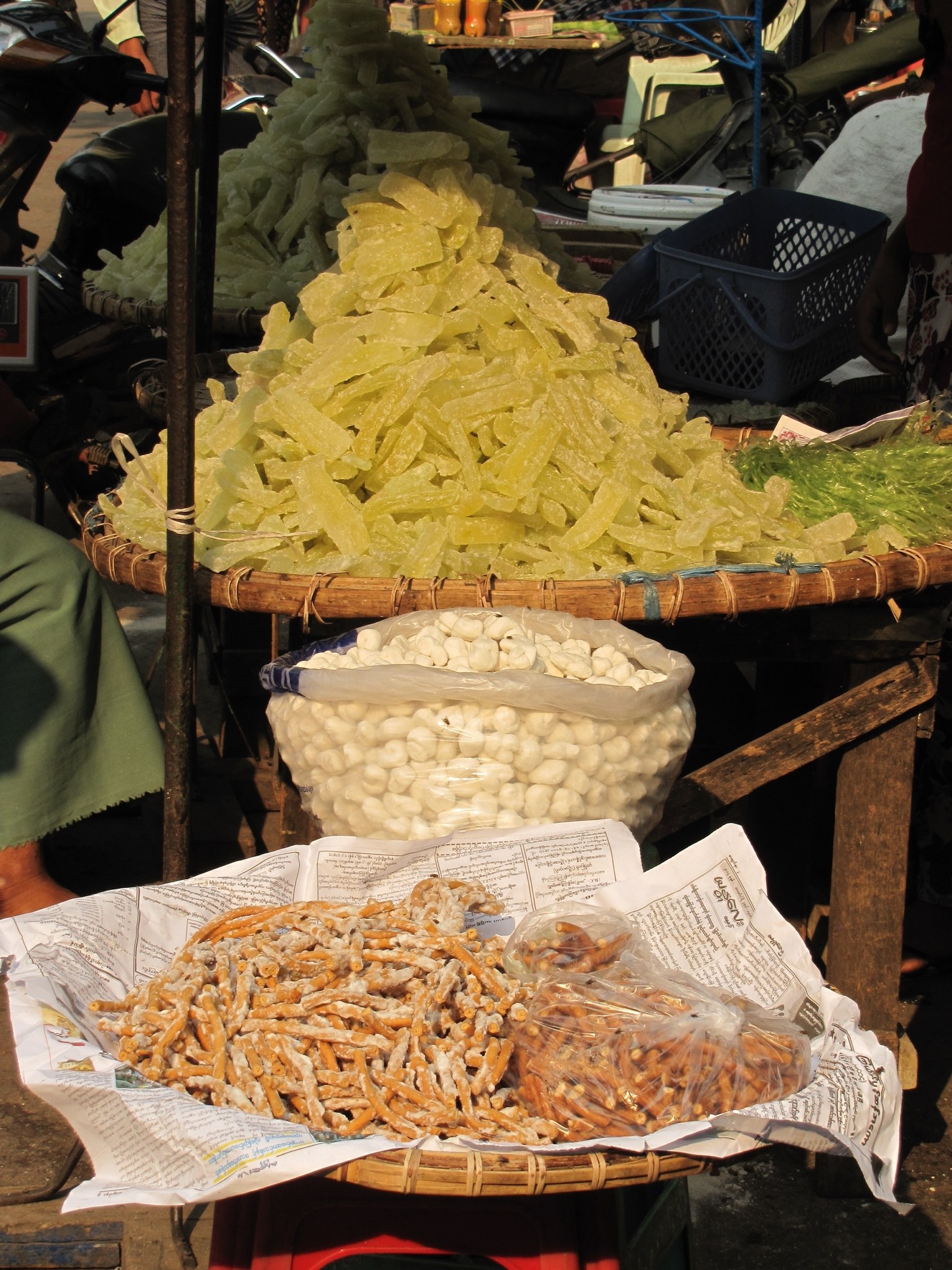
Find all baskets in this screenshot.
[79,272,297,337]
[596,227,673,382]
[135,343,258,423]
[653,189,891,406]
[307,978,840,1196]
[77,423,952,621]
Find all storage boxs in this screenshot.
[652,187,892,402]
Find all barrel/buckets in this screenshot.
[587,185,742,346]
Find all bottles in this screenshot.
[463,0,488,36]
[434,1,461,35]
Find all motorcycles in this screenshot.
[461,13,846,283]
[1,1,307,541]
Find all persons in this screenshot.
[86,0,277,116]
[851,1,952,404]
[0,506,169,918]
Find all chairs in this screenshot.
[600,0,806,187]
